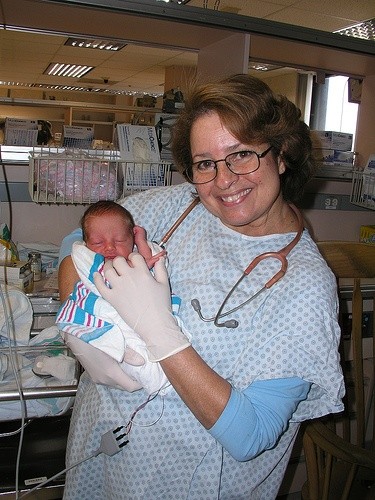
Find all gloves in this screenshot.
[59,329,145,393]
[93,240,192,364]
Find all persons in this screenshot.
[71,201,169,395]
[59,74,345,500]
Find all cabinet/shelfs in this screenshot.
[0,98,162,146]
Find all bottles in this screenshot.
[27,251,42,281]
[19,264,35,293]
[174,86,186,113]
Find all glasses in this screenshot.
[182,144,273,185]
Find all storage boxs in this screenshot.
[359,225,375,244]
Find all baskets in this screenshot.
[349,170,375,211]
[28,143,173,207]
[359,225,375,245]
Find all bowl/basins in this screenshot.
[136,97,156,107]
